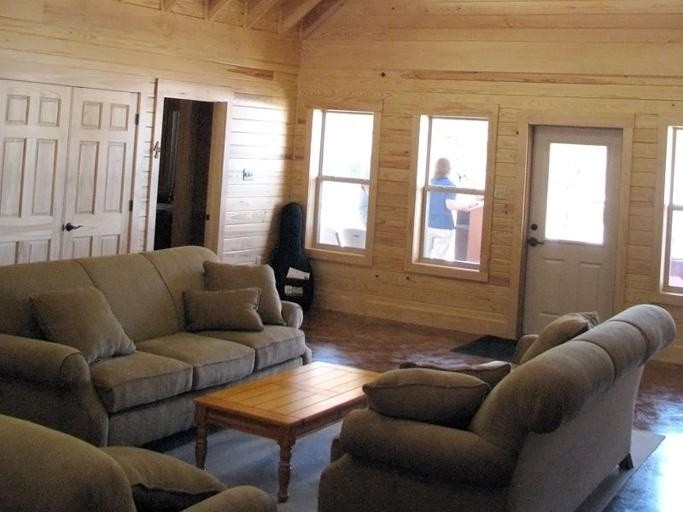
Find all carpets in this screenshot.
[136,420,666,512]
[449,333,521,365]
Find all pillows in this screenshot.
[179,287,265,332]
[24,284,138,366]
[201,260,288,326]
[94,443,229,511]
[360,367,493,427]
[397,359,511,389]
[519,310,600,365]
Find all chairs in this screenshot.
[0,412,277,511]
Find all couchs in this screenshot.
[315,302,678,512]
[0,244,312,458]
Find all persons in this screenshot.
[426,158,469,257]
